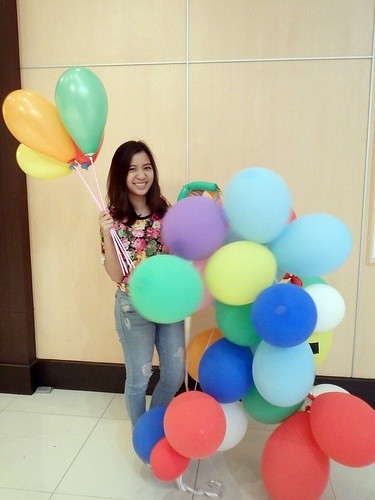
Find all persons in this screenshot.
[98,141,205,469]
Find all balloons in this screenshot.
[2,67,108,180]
[128,166,375,500]
